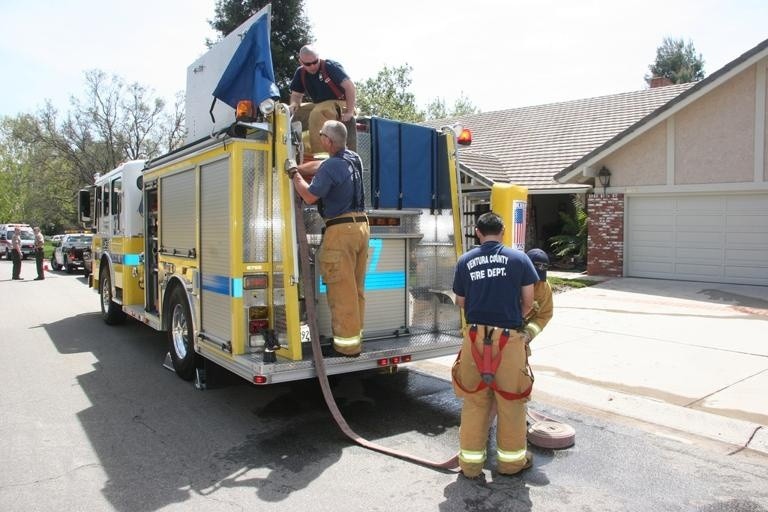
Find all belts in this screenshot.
[326,216,367,227]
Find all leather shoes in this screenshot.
[12,276,24,280]
[33,276,45,280]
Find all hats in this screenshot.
[526,248,549,282]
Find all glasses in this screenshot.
[319,130,329,139]
[301,58,318,67]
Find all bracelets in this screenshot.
[290,171,298,178]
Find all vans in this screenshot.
[0,224,35,261]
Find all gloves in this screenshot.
[285,159,298,178]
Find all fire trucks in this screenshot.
[77,98,475,391]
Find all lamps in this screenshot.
[596,165,612,197]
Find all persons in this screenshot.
[33,226,45,280]
[289,45,355,154]
[285,119,373,356]
[11,227,24,280]
[454,210,552,479]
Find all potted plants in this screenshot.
[644,32,706,87]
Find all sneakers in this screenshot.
[321,345,362,357]
[499,451,535,476]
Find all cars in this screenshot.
[46,234,96,278]
